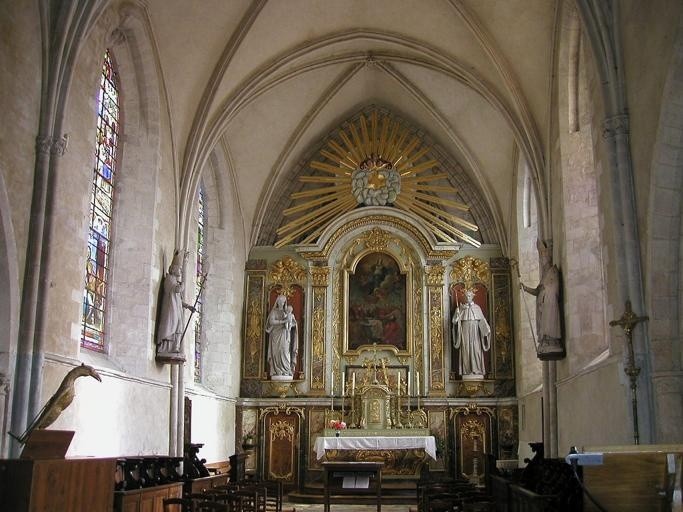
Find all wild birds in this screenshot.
[18,362,104,449]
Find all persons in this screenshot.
[264,296,298,375]
[520,238,562,345]
[452,291,490,374]
[156,249,196,353]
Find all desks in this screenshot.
[320,461,383,511]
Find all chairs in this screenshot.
[161,477,295,512]
[408,477,494,511]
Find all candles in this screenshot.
[397,371,419,395]
[329,372,356,396]
